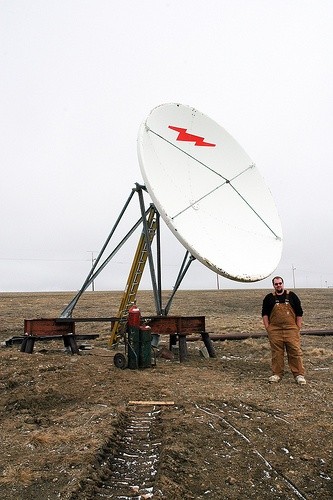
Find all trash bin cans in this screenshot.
[128,324,154,368]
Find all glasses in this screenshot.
[274,282,283,286]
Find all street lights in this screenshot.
[292,267,296,288]
[92,258,96,290]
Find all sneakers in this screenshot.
[295,375,307,386]
[267,375,282,383]
